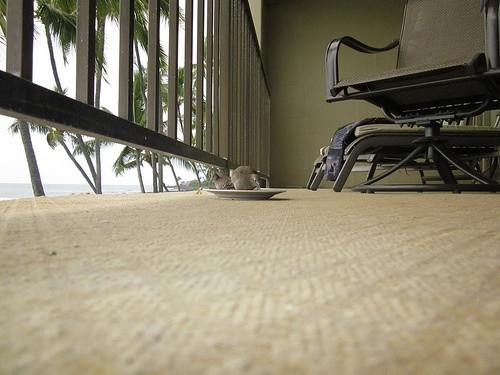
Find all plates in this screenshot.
[202,189,287,201]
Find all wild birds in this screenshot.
[230,166,271,190]
[209,173,233,189]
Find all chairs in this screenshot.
[306,123,500,191]
[320,0,500,195]
